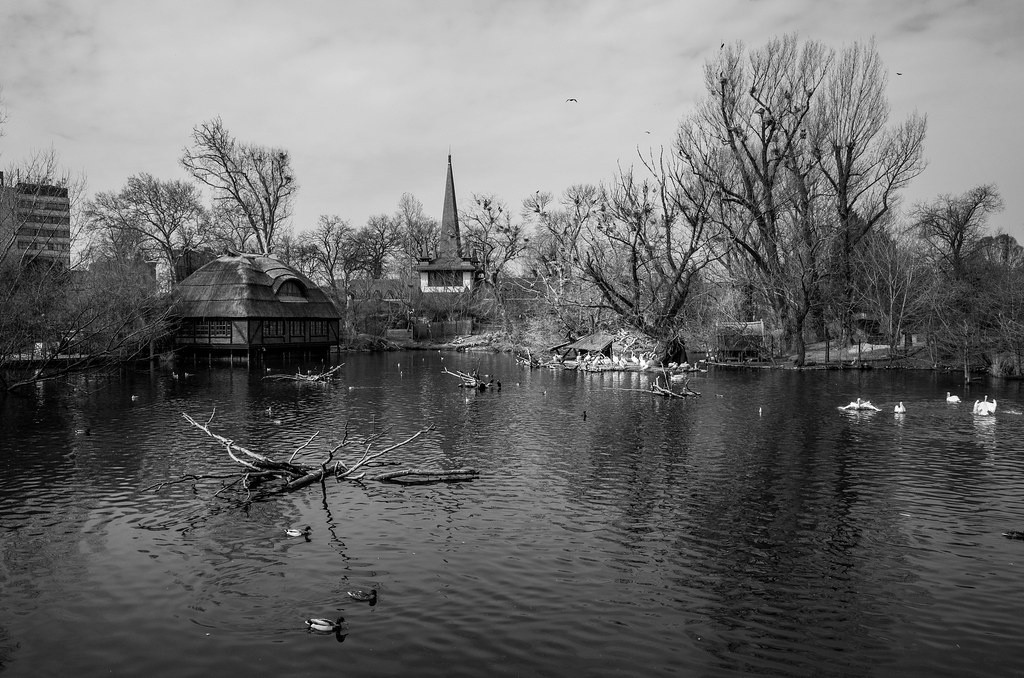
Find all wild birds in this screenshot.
[347,589,377,600]
[74,428,91,433]
[580,411,587,421]
[843,398,906,413]
[284,526,313,537]
[946,392,997,415]
[304,616,349,632]
[171,372,194,379]
[130,394,139,398]
